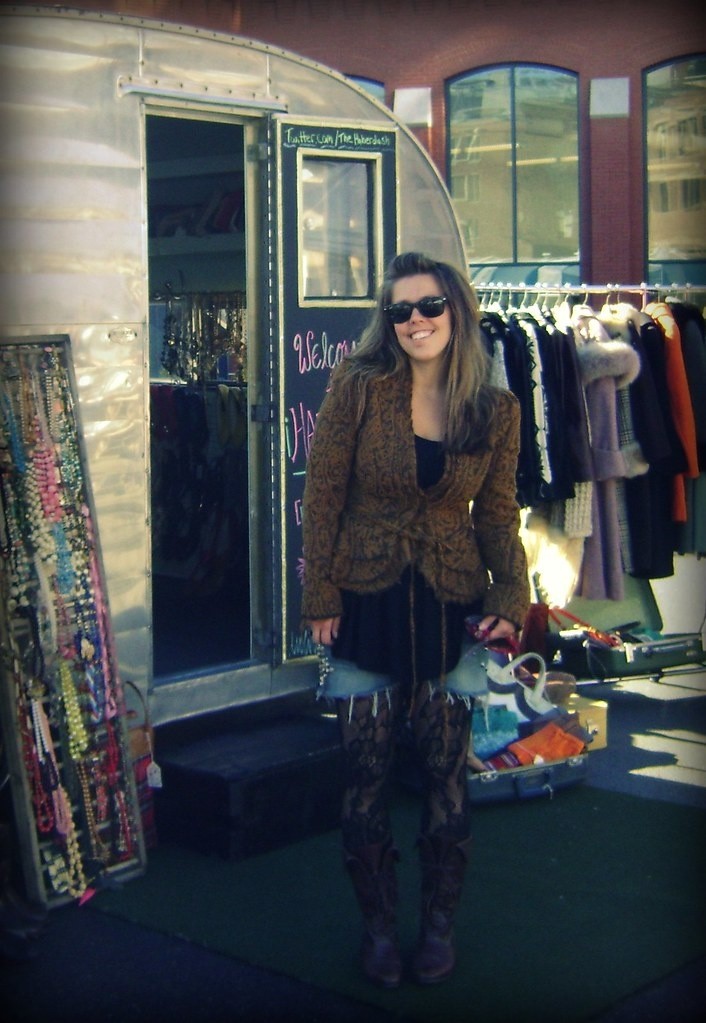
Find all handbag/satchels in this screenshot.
[480,652,560,723]
[119,678,162,828]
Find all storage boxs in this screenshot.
[548,576,706,679]
[470,752,590,802]
[559,695,609,752]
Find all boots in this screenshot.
[342,835,403,986]
[408,838,471,986]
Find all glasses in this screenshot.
[383,295,450,323]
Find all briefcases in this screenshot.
[461,743,590,801]
[559,621,704,676]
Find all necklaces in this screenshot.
[0,343,133,898]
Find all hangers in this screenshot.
[476,284,677,313]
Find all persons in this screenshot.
[301,253,531,987]
